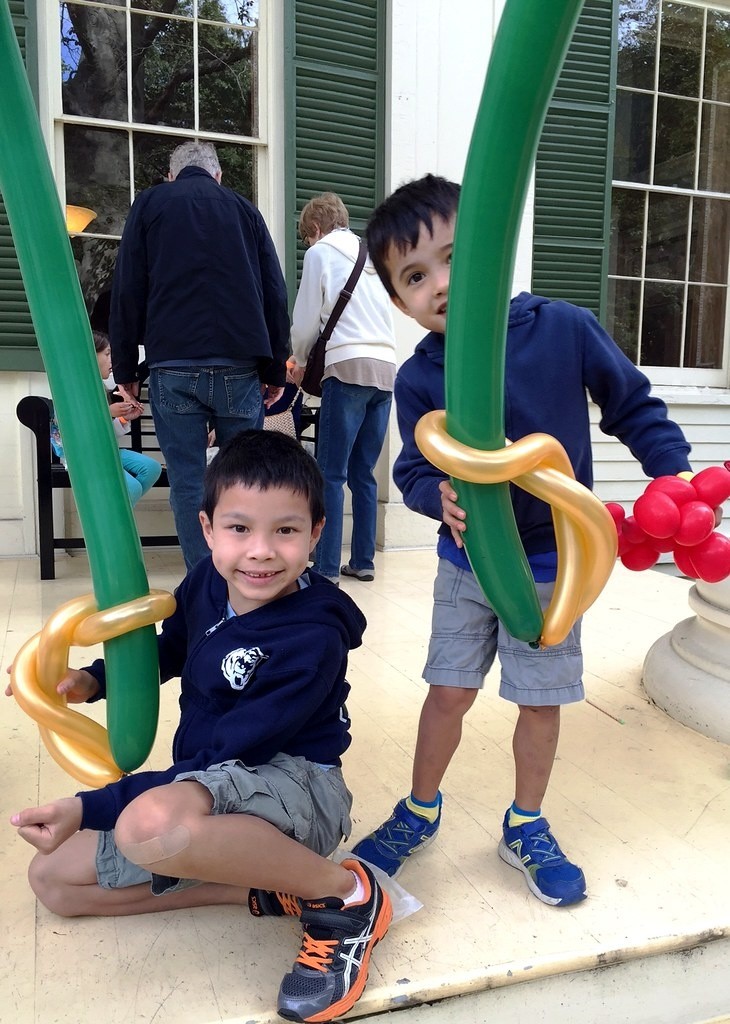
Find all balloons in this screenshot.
[0,0,177,790]
[605,460,730,583]
[413,0,617,649]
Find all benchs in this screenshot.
[17,368,323,580]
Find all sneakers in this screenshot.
[325,577,340,587]
[351,791,443,881]
[341,564,374,581]
[277,858,393,1022]
[249,888,304,918]
[498,808,588,906]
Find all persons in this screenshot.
[352,173,724,906]
[53,330,163,508]
[8,428,393,1024]
[288,193,397,589]
[108,140,292,573]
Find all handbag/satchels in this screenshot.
[300,337,326,397]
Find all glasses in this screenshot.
[301,234,309,248]
